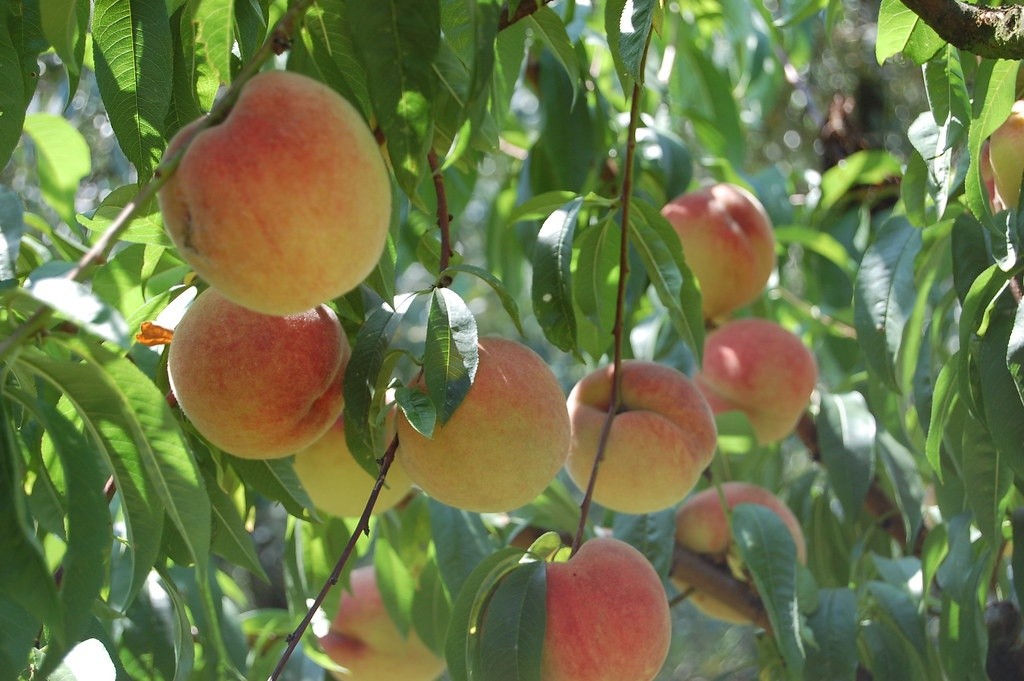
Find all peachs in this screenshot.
[149,72,1024,681]
